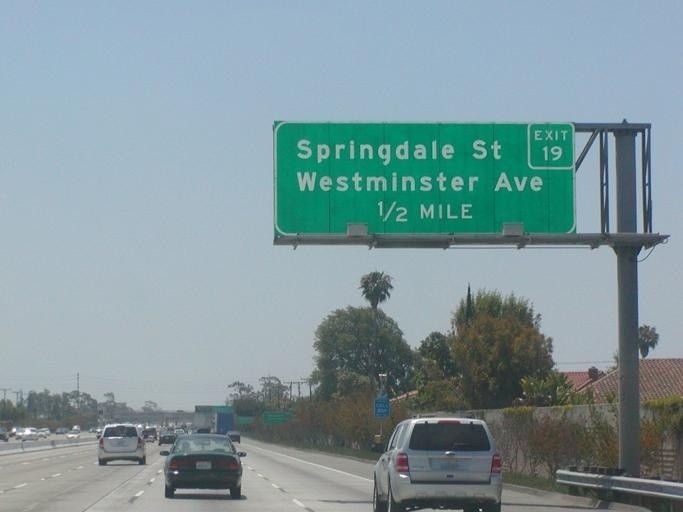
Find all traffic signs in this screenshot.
[262,412,294,424]
[373,398,390,417]
[273,120,576,235]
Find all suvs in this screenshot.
[371,417,505,512]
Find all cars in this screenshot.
[0,420,246,499]
